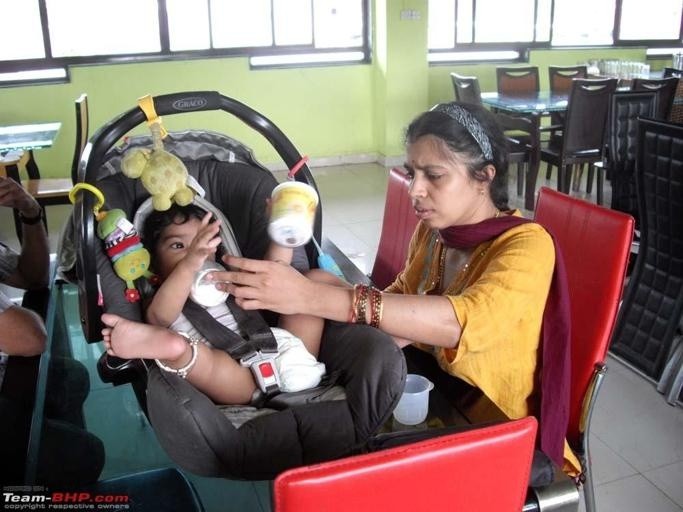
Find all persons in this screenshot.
[1,178,105,490]
[100,204,415,397]
[197,101,584,482]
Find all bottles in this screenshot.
[185,262,231,309]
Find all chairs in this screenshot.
[522,186,635,512]
[549,66,587,135]
[368,168,420,290]
[480,86,682,210]
[524,78,618,195]
[450,72,531,194]
[270,416,539,512]
[496,66,563,139]
[587,65,663,86]
[606,68,683,407]
[21,94,89,237]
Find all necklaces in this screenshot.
[424,209,501,299]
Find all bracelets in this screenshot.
[350,282,382,328]
[16,207,44,227]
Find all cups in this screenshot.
[390,373,436,426]
[264,181,326,250]
[671,53,682,79]
[600,59,650,77]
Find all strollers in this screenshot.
[71,91,407,481]
